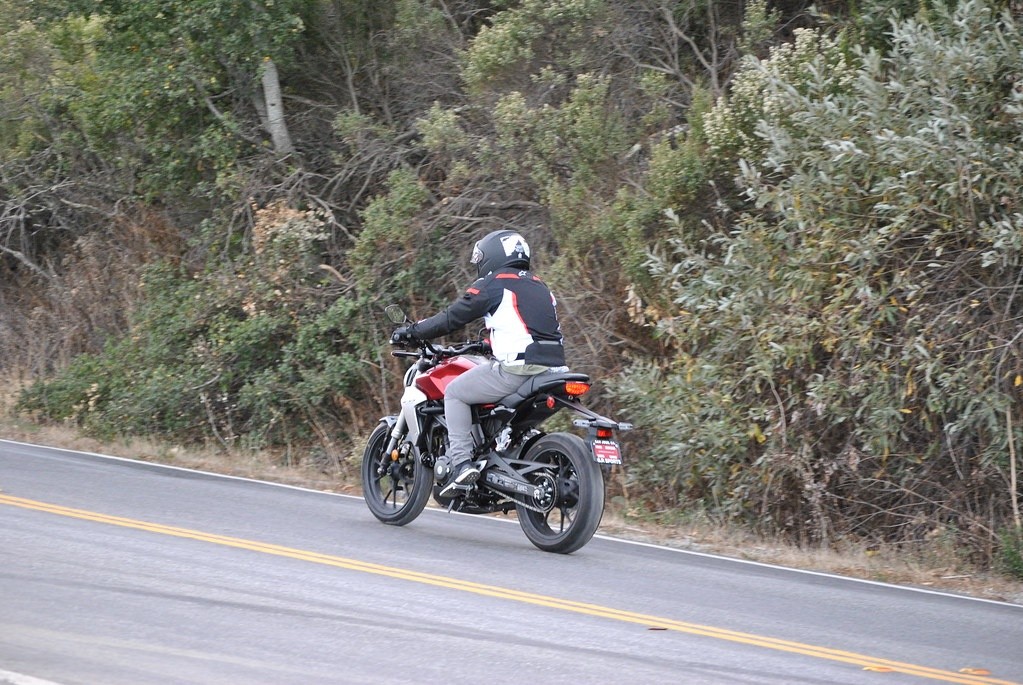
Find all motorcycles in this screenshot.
[358,302,637,554]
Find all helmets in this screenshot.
[470,230,531,277]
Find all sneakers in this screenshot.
[439,462,480,497]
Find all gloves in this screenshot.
[390,325,412,348]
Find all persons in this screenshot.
[391,229,570,497]
